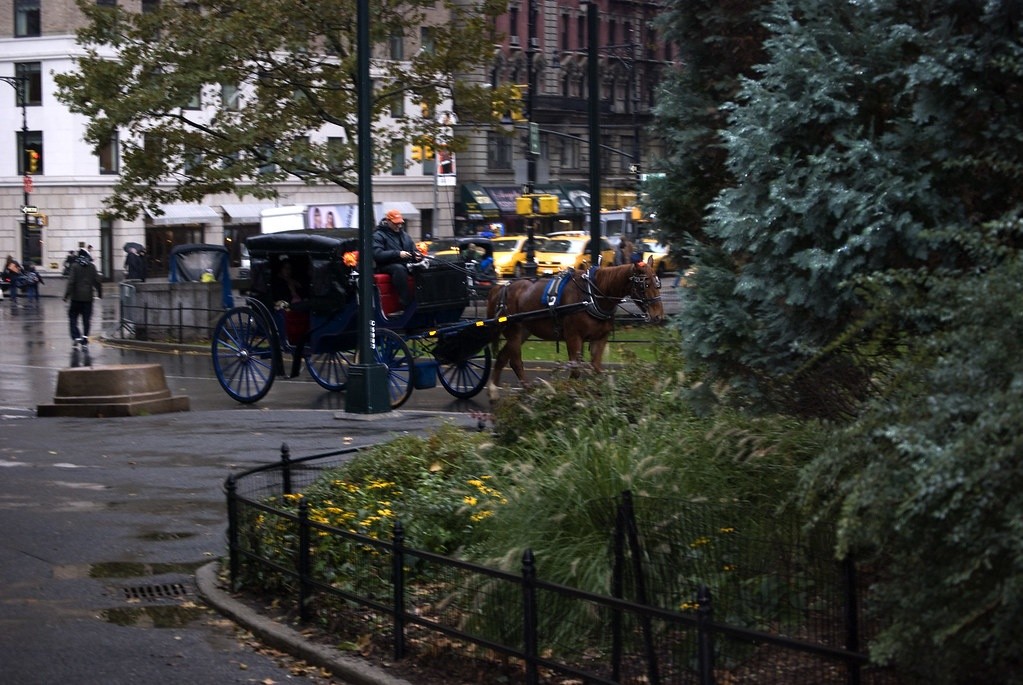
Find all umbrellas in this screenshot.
[123,243,143,253]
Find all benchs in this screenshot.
[251,287,310,345]
[374,271,415,318]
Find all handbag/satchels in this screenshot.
[8,263,21,274]
[63,266,70,275]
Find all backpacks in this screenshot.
[28,273,39,286]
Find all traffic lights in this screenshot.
[24,142,42,173]
[35,214,48,226]
[412,135,436,161]
[491,87,524,121]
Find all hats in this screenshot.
[6,255,13,259]
[77,248,92,261]
[387,209,404,224]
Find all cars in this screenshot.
[415,232,680,277]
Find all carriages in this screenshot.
[211,228,664,413]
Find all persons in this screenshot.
[616,237,685,301]
[376,210,427,311]
[311,207,335,227]
[2,242,147,349]
[462,243,492,280]
[275,257,309,340]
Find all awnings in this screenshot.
[134,199,284,226]
[459,179,647,219]
[383,201,421,220]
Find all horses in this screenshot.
[487,237,664,405]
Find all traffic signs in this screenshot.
[19,205,37,213]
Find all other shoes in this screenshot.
[82,338,88,344]
[74,340,81,346]
